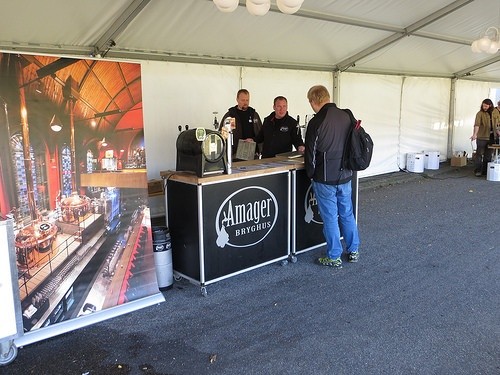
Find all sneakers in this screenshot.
[315,257,343,269]
[348,248,359,262]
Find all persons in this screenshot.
[470,99,500,176]
[218,89,261,162]
[304,85,361,269]
[244,96,305,158]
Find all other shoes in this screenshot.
[475,172,482,176]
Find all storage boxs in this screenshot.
[159,151,359,296]
[451,156,467,167]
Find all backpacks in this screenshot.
[341,109,373,171]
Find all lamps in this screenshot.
[470,26,500,54]
[50,113,63,132]
[213,0,303,17]
[36,83,44,94]
[103,138,108,147]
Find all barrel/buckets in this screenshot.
[151,226,174,291]
